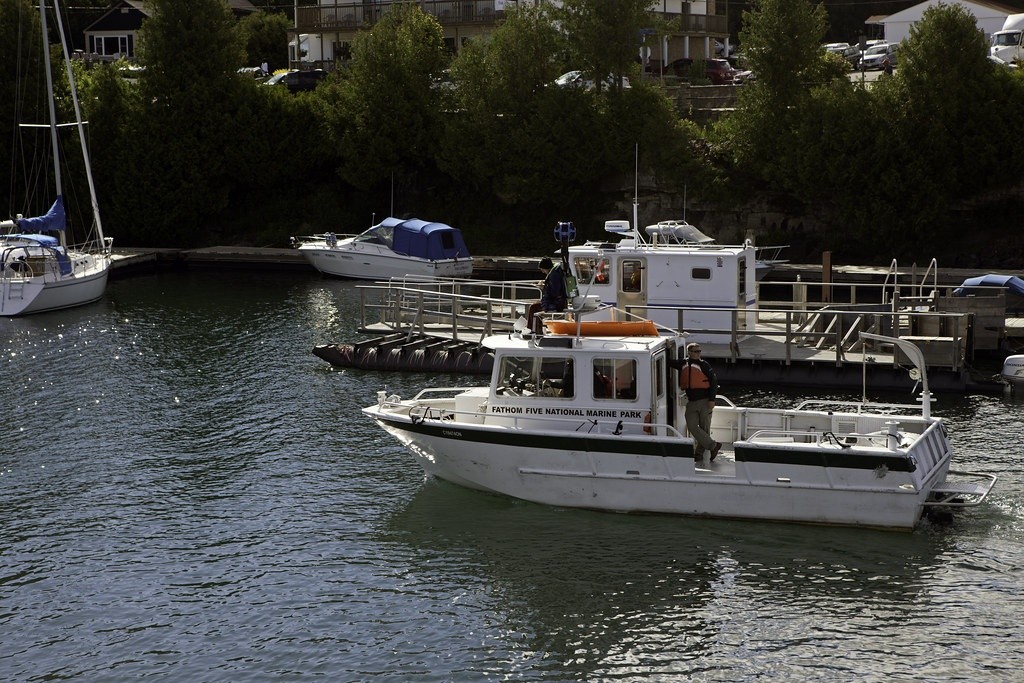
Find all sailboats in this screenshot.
[0,0,116,317]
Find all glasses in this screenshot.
[691,350,702,353]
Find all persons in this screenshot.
[261,59,268,73]
[12,256,15,261]
[547,361,574,397]
[523,257,567,339]
[881,59,893,75]
[668,344,720,460]
[993,47,998,57]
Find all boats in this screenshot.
[291,214,476,284]
[930,272,1024,316]
[357,259,999,536]
[551,182,791,295]
[998,352,1024,386]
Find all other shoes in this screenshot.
[710,442,722,461]
[523,331,543,339]
[694,452,703,462]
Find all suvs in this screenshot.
[649,57,735,87]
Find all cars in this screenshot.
[713,38,904,87]
[262,69,330,91]
[533,70,633,96]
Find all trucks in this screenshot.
[985,12,1024,71]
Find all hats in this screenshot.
[539,258,553,269]
